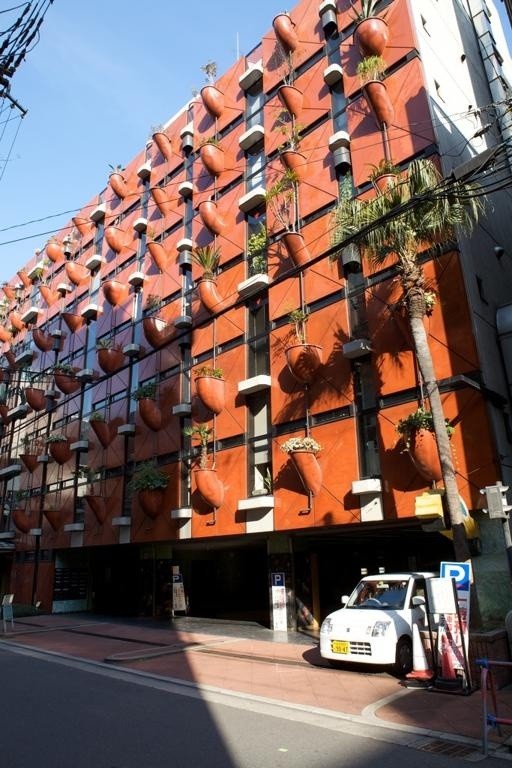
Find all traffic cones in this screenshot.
[435,628,462,688]
[403,620,435,682]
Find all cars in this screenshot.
[314,567,442,679]
